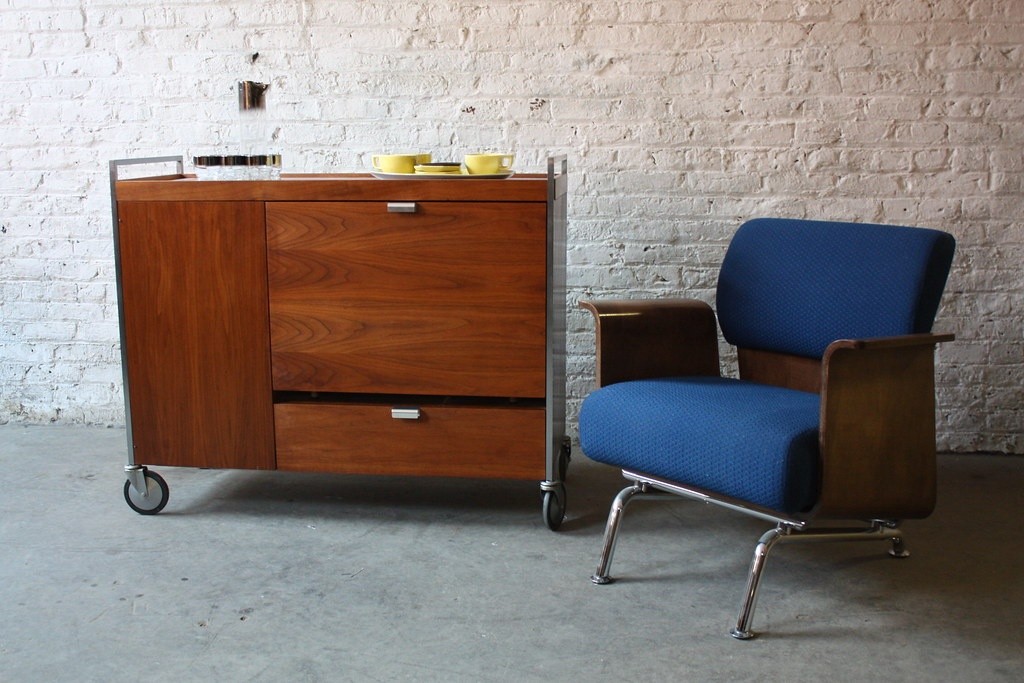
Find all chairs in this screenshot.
[578,210,956,642]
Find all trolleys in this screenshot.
[109,154,573,530]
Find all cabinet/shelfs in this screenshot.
[97,155,571,530]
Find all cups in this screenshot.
[415,154,432,164]
[193,155,282,182]
[464,153,516,174]
[372,155,415,174]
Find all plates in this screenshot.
[413,163,461,173]
